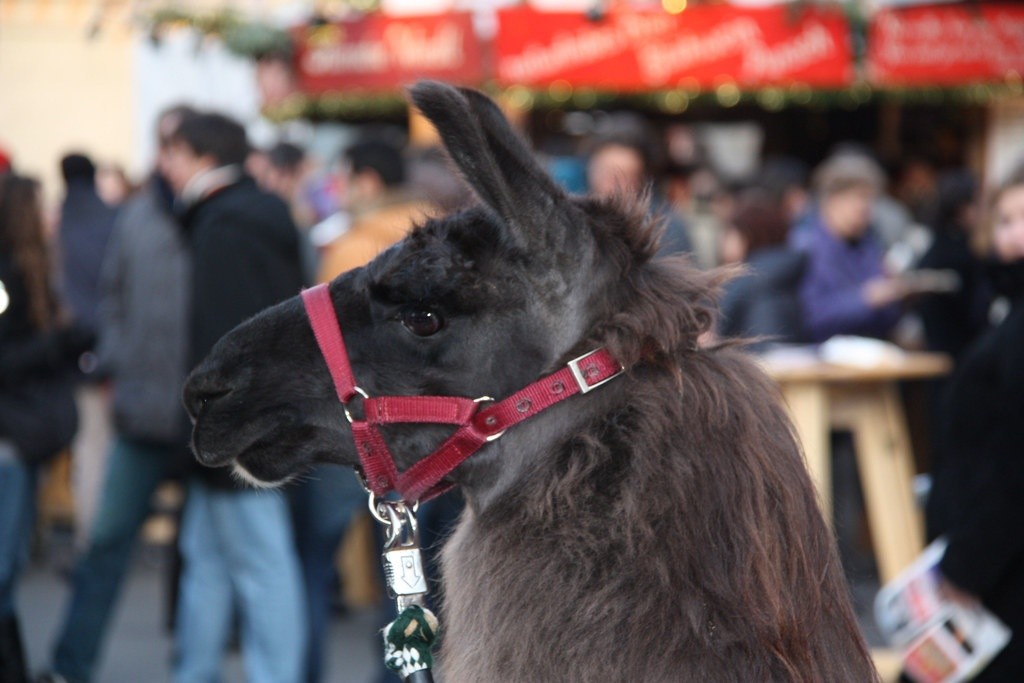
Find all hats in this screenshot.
[815,153,881,197]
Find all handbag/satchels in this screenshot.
[713,250,806,353]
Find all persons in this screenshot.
[582,117,1024,683]
[0,107,482,683]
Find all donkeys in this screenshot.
[176,80,890,682]
